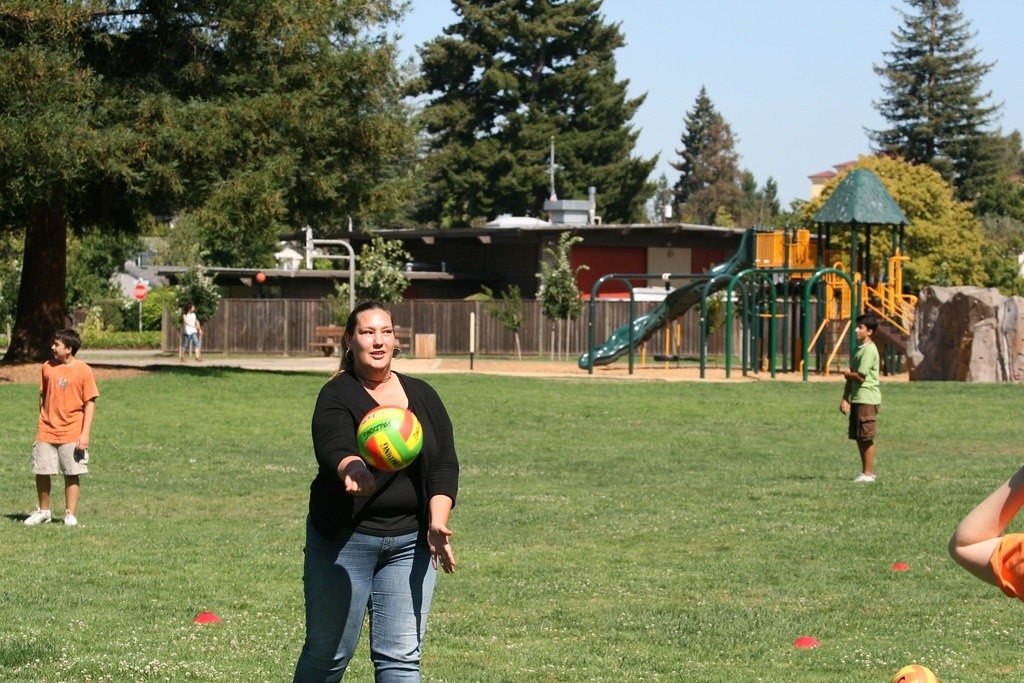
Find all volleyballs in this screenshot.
[356,405,425,471]
[890,664,939,683]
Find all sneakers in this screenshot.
[64,510,77,526]
[23,505,51,526]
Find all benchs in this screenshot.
[308,326,413,357]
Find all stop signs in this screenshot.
[133,284,148,300]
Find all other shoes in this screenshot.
[853,472,876,482]
[180,357,185,362]
[195,358,202,362]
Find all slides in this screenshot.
[578,226,774,370]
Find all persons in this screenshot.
[294,301,460,683]
[22,328,99,526]
[948,463,1024,604]
[840,313,881,483]
[181,303,203,362]
